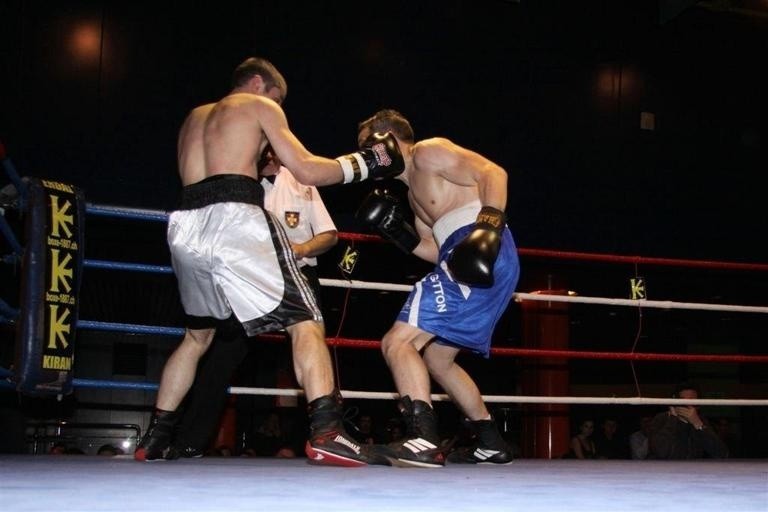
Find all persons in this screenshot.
[258,413,296,454]
[352,413,402,446]
[49,444,126,455]
[570,386,740,460]
[447,406,522,456]
[133,55,404,474]
[356,111,528,473]
[175,142,342,456]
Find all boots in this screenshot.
[305,388,399,468]
[386,394,515,469]
[134,405,204,462]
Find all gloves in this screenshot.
[333,131,505,289]
